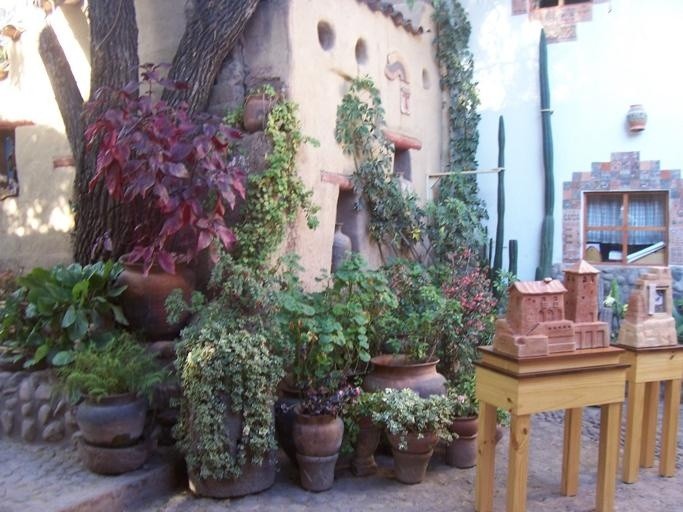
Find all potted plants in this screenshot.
[168,302,281,495]
[282,253,479,494]
[61,334,165,473]
[241,83,283,131]
[78,61,246,338]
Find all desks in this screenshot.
[473,340,683,511]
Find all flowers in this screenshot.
[427,244,503,365]
